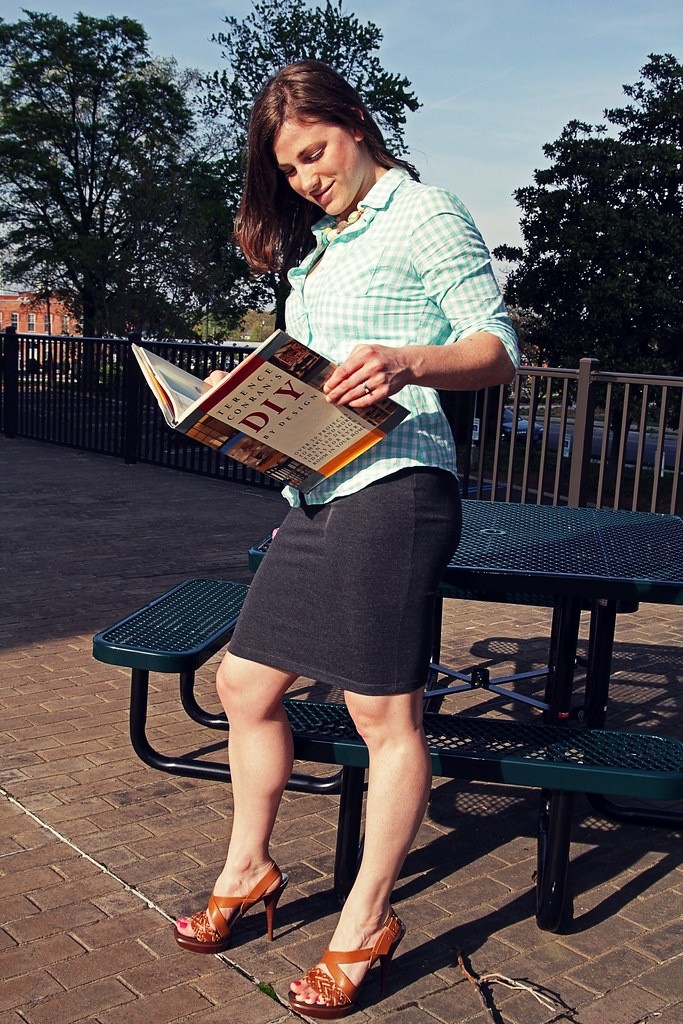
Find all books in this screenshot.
[132,330,410,494]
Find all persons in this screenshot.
[173,60,522,1019]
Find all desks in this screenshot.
[248,496,683,930]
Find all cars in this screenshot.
[502,407,544,446]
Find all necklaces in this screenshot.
[322,201,365,244]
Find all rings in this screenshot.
[362,382,371,394]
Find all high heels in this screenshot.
[287,908,406,1019]
[174,862,289,954]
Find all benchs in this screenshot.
[95,532,683,930]
